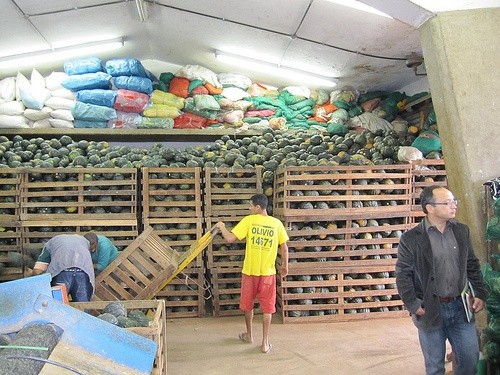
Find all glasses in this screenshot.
[426,199,461,206]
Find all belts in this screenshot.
[439,295,461,304]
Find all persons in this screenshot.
[34,234,95,302]
[216,193,289,353]
[84,233,121,284]
[394,186,487,375]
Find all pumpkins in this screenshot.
[0,127,447,327]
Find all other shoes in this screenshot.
[239,330,250,344]
[264,343,272,354]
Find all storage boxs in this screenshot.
[0,160,448,375]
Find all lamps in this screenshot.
[135,0,148,22]
[214,49,338,87]
[0,38,124,64]
